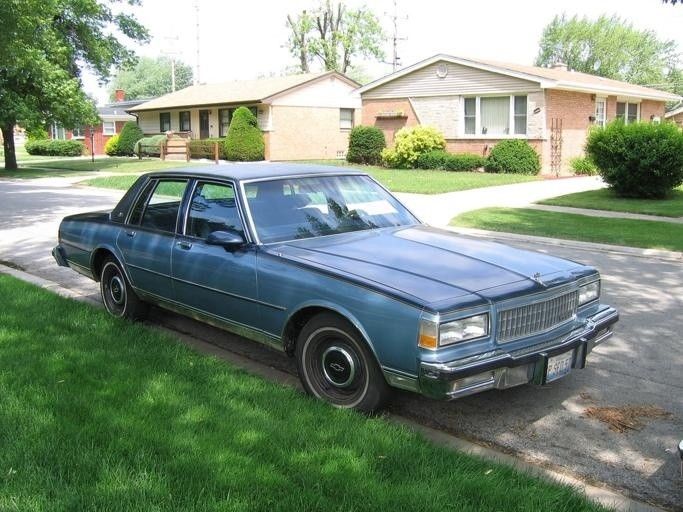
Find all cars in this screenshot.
[50,164,619,418]
